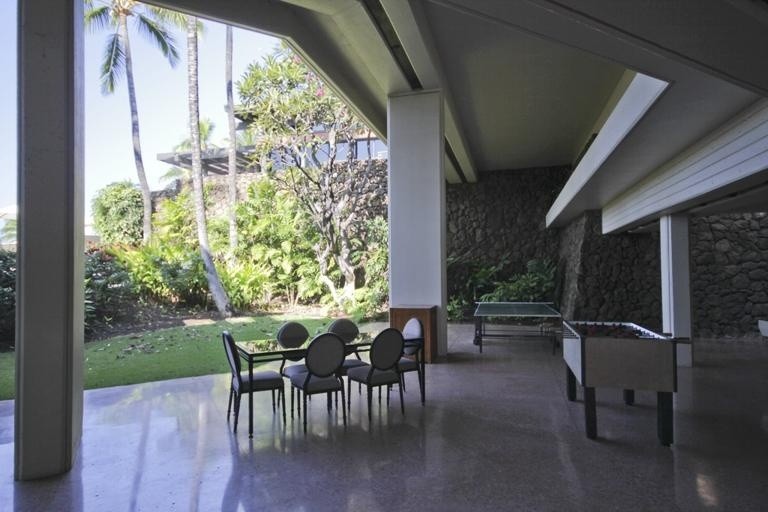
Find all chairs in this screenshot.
[223,317,425,439]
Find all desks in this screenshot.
[474,302,561,355]
